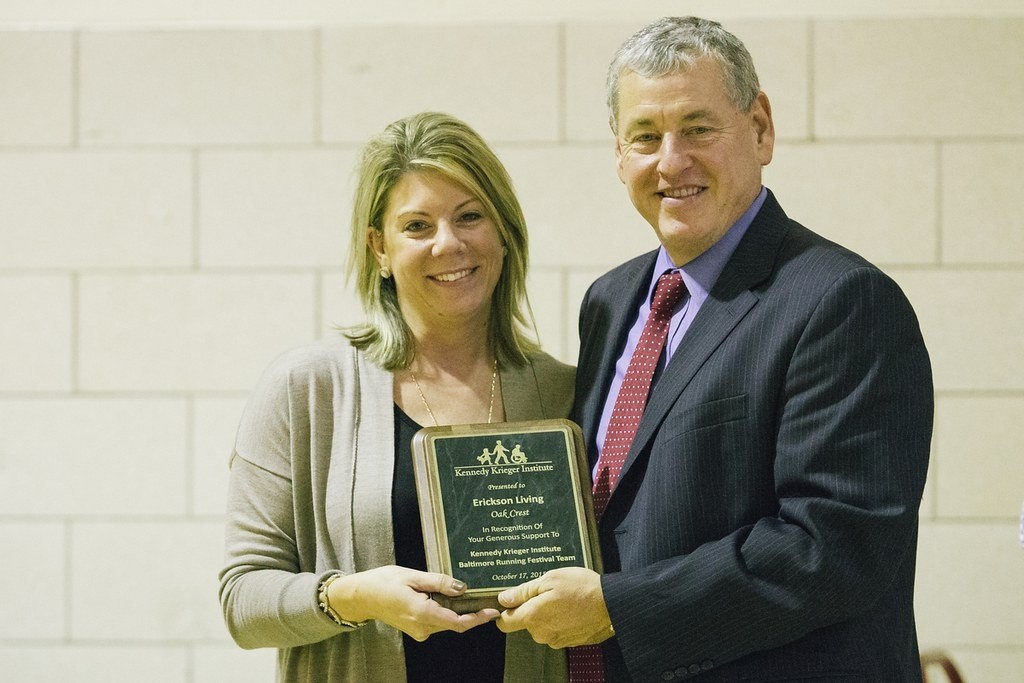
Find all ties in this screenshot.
[566,271,684,683]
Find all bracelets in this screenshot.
[610,625,615,634]
[318,573,370,629]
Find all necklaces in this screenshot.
[400,354,499,439]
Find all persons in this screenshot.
[498,15,936,683]
[214,114,581,683]
